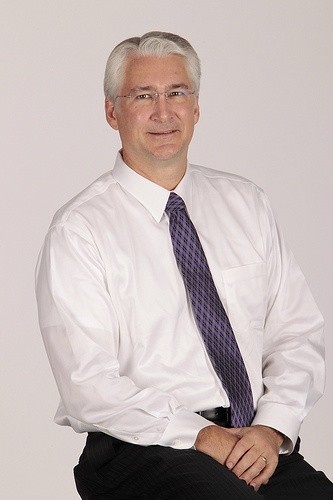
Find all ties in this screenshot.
[158,192,256,429]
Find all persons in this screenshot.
[35,32,333,499]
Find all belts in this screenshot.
[194,408,231,424]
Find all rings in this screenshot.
[261,456,267,463]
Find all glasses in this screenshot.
[112,85,196,106]
